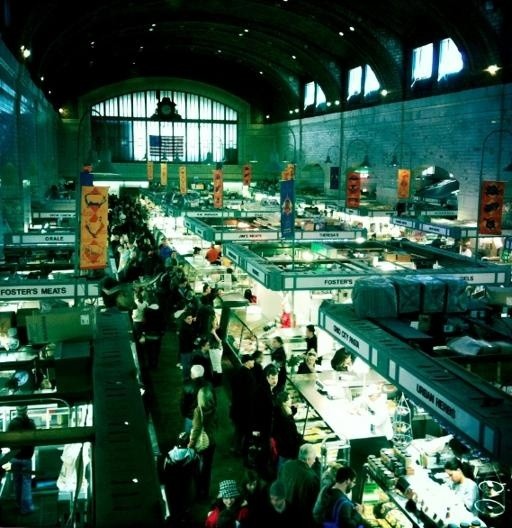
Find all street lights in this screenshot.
[68,103,117,306]
[245,140,306,202]
[325,145,342,206]
[345,137,373,211]
[389,140,412,221]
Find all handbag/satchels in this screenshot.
[194,427,210,453]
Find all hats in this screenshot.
[17,405,27,415]
[241,354,253,363]
[269,480,284,497]
[191,364,204,378]
[217,480,241,499]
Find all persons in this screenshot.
[133,295,223,387]
[4,404,39,514]
[164,322,370,527]
[361,369,395,443]
[99,192,227,304]
[441,458,481,518]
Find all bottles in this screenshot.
[367,444,411,489]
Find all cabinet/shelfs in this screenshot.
[0,200,512,528]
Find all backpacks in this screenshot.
[241,425,266,469]
